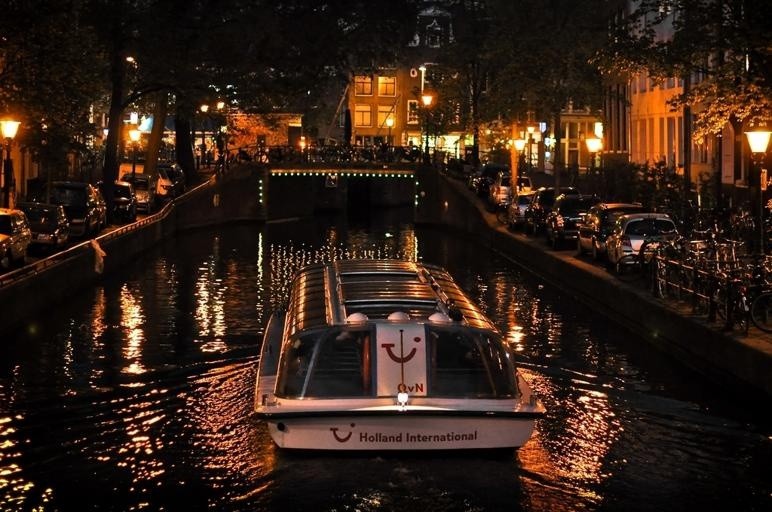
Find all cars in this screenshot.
[0,163,184,273]
[446,152,682,275]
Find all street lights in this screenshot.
[0,117,22,208]
[129,128,142,191]
[513,137,525,192]
[585,134,602,206]
[420,91,435,166]
[740,118,772,320]
[200,102,207,165]
[385,117,394,145]
[216,101,225,158]
[526,122,536,167]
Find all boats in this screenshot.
[254,258,548,458]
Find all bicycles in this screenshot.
[653,211,772,336]
